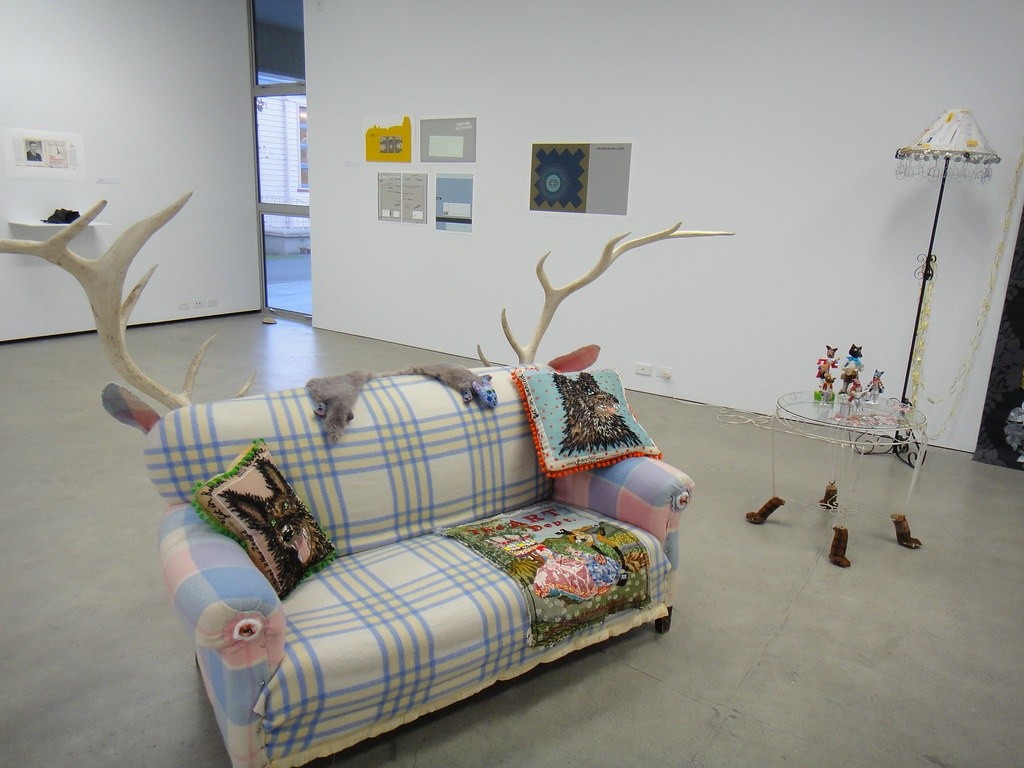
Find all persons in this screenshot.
[26,141,44,163]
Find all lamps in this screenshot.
[855,112,1000,470]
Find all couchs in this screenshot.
[139,364,697,768]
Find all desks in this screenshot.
[743,391,921,568]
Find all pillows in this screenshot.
[192,439,341,600]
[509,366,667,477]
